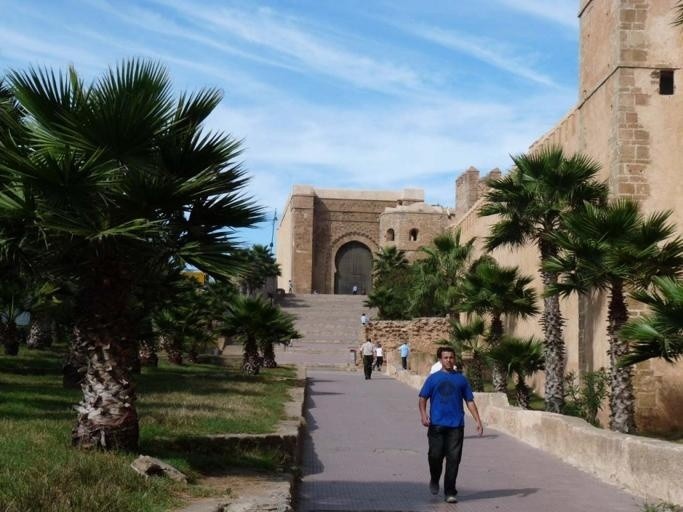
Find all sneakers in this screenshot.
[429,480,439,494]
[444,494,457,502]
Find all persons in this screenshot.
[375,343,384,371]
[429,346,458,374]
[288,279,293,293]
[351,284,358,295]
[360,337,376,380]
[418,346,484,503]
[397,342,410,370]
[360,312,368,326]
